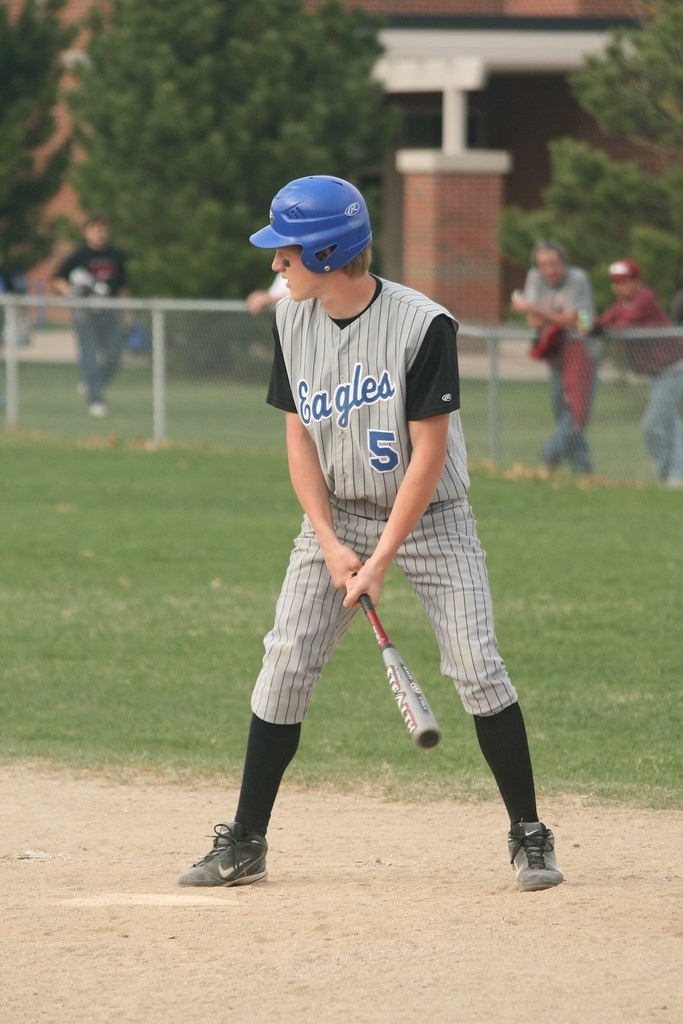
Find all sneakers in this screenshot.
[177,820,268,886]
[507,822,564,891]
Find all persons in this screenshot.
[47,218,134,419]
[179,174,683,890]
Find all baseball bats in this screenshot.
[352,572,442,749]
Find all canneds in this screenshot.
[578,309,594,335]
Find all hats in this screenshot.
[605,259,639,282]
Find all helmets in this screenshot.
[249,175,372,273]
